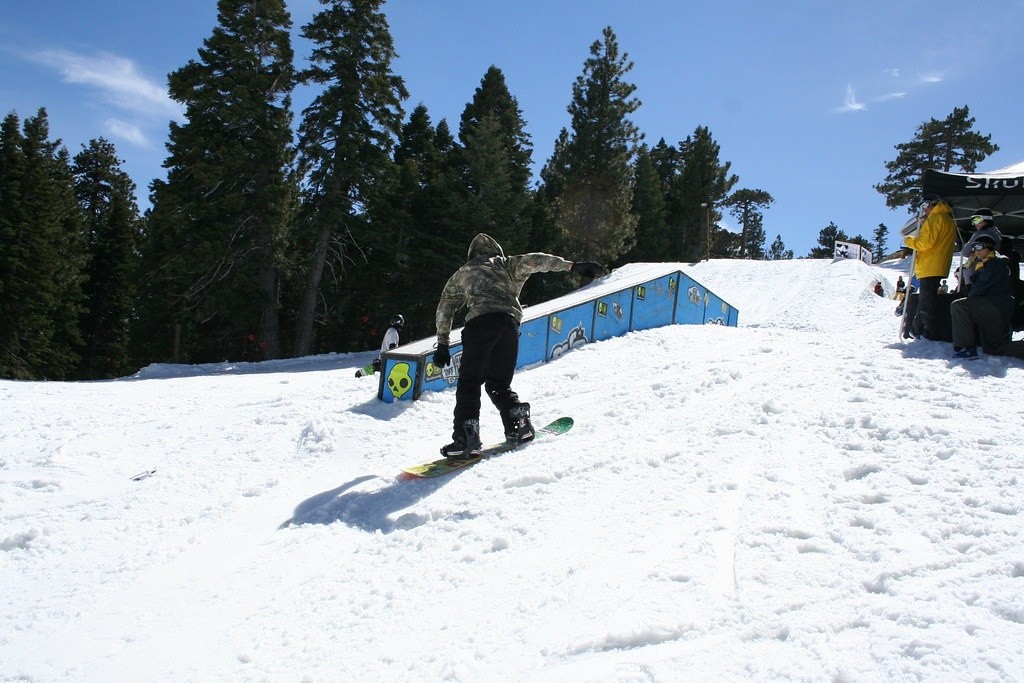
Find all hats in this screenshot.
[974,207,993,224]
[974,234,996,251]
[923,194,941,207]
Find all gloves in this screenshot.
[433,344,450,368]
[570,261,607,278]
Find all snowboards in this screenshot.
[399,416,574,479]
[355,361,381,379]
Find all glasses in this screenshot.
[954,272,960,277]
[920,199,939,210]
[971,215,993,225]
[972,241,996,252]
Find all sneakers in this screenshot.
[440,441,483,459]
[505,424,535,443]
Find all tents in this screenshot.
[898,159,1024,336]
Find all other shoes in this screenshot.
[953,347,978,361]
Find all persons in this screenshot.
[433,233,609,458]
[950,235,1015,361]
[379,314,404,359]
[940,280,948,293]
[962,207,1002,296]
[875,282,883,297]
[904,194,957,339]
[912,272,919,292]
[893,276,905,300]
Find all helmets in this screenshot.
[390,314,404,331]
